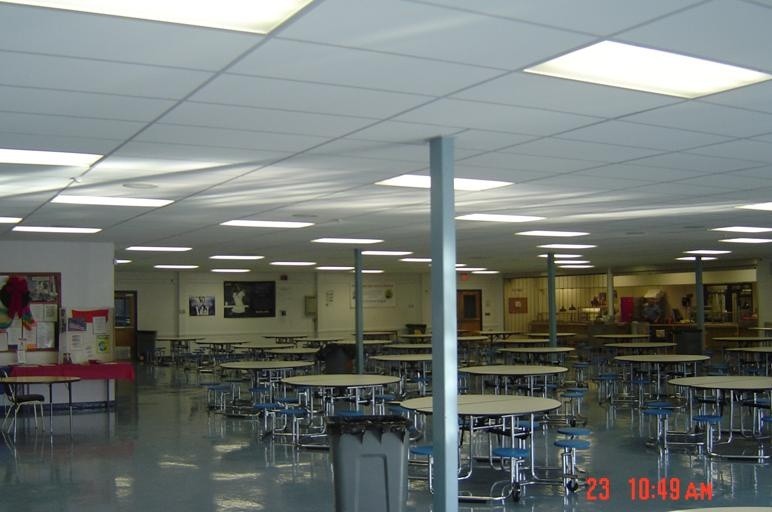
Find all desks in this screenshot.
[10,363,135,411]
[0,377,79,435]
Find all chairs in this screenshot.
[0,369,48,443]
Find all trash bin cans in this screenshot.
[136,330,157,361]
[406,323,426,352]
[323,415,413,512]
[670,328,703,355]
[324,343,355,396]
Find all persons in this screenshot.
[232,282,248,315]
[639,297,662,324]
